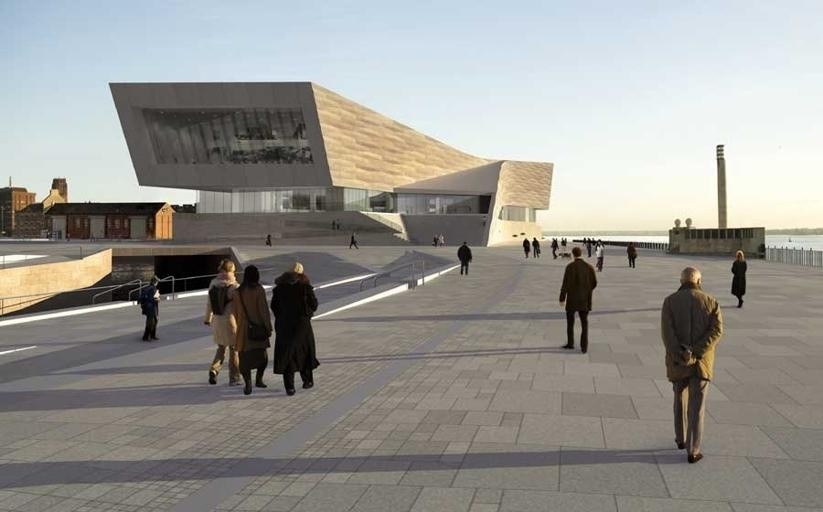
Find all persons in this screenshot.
[232,265,272,394]
[350,232,358,249]
[265,235,271,248]
[458,242,472,275]
[141,275,160,342]
[434,232,448,246]
[660,267,723,463]
[560,247,597,353]
[483,214,487,226]
[523,237,637,271]
[270,262,318,396]
[731,250,747,308]
[332,218,340,230]
[205,259,242,386]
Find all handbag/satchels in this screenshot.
[247,322,269,342]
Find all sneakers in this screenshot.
[209,370,218,384]
[229,380,243,386]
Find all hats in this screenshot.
[289,262,303,275]
[150,277,159,281]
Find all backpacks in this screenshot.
[141,286,153,314]
[209,284,235,315]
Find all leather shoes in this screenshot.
[286,386,296,395]
[256,382,267,388]
[303,380,313,389]
[687,453,702,463]
[675,439,684,449]
[244,386,252,395]
